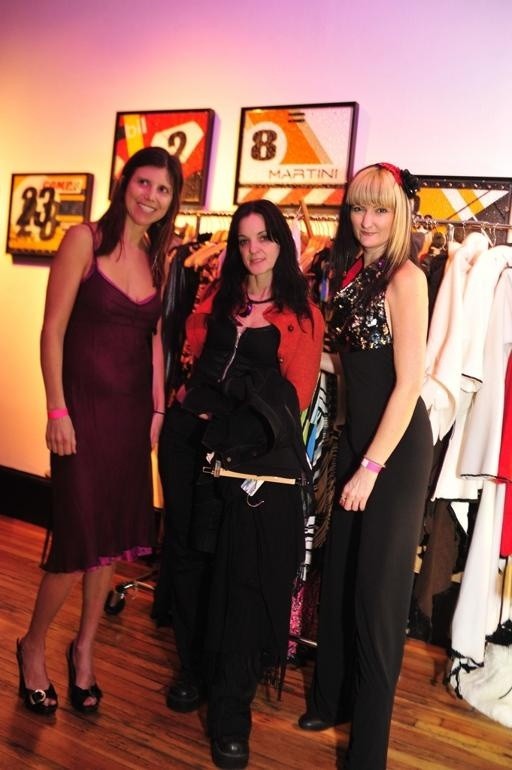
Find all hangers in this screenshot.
[162,207,508,275]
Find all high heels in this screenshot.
[66,641,102,712]
[17,637,58,715]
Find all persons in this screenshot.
[159,198,326,769]
[298,162,435,770]
[15,146,185,715]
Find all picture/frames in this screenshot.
[233,101,360,210]
[107,106,215,207]
[5,173,94,260]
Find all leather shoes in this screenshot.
[166,670,206,712]
[211,736,249,770]
[299,712,350,729]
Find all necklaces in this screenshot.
[238,289,273,318]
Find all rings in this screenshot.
[339,495,347,501]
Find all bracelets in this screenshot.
[360,455,383,473]
[46,407,69,420]
[152,410,168,417]
